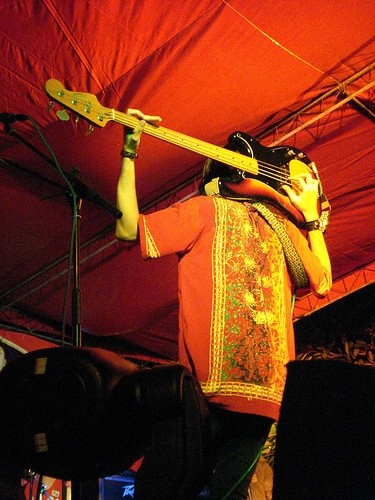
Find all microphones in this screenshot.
[67,180,123,219]
[0,112,28,123]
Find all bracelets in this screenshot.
[120,150,138,158]
[304,219,319,232]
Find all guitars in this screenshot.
[44,77,331,232]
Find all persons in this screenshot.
[246,453,274,500]
[113,108,333,500]
